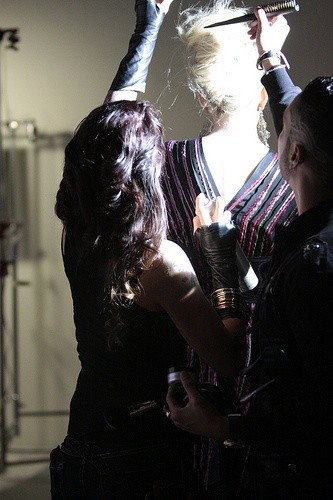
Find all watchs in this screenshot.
[223,413,242,451]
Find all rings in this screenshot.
[165,410,171,420]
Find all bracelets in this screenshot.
[256,49,282,71]
[210,288,244,311]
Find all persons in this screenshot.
[158,8,300,500]
[162,4,333,500]
[49,0,247,500]
